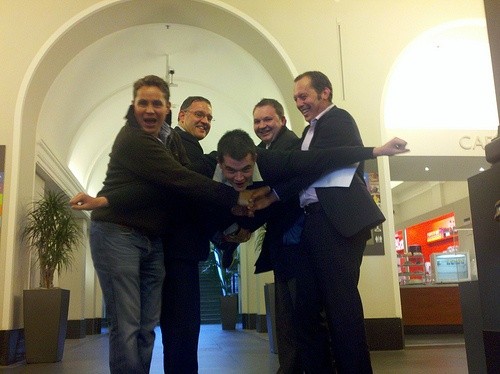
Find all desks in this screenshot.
[400,282,463,335]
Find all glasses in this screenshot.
[185,110,215,122]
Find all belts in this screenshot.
[304,202,321,215]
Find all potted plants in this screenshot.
[22,190,86,364]
[255,222,278,354]
[201,240,241,330]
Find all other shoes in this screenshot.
[222,251,234,269]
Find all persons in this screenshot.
[160,97,262,374]
[293,72,386,374]
[89,75,270,374]
[70,129,410,269]
[253,99,336,374]
